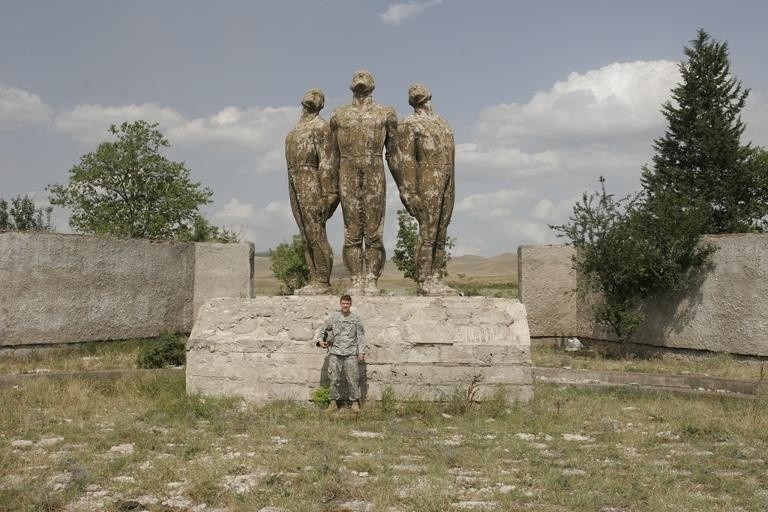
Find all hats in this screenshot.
[325,330,333,346]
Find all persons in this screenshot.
[285,87,342,297]
[328,70,417,295]
[314,295,368,413]
[398,83,460,298]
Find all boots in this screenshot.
[351,401,361,412]
[327,399,337,411]
